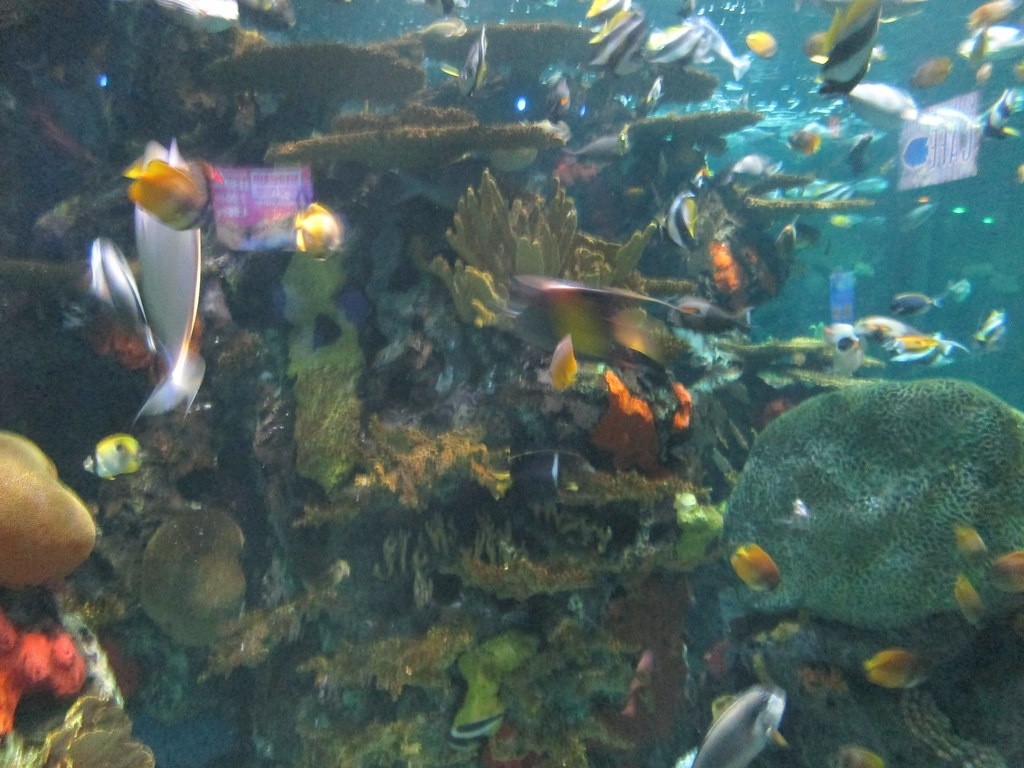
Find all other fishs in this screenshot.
[81,2,1024,768]
